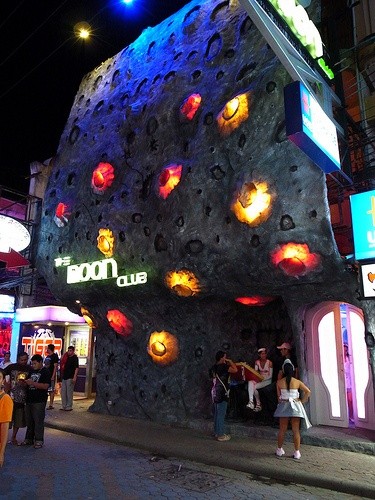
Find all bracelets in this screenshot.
[34,382,38,387]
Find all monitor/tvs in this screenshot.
[359,262,375,300]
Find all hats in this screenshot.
[276,342,291,349]
[282,358,294,372]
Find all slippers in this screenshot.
[20,442,30,445]
[34,444,42,449]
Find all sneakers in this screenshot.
[246,402,263,412]
[276,447,302,459]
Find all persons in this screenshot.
[20,354,52,449]
[271,342,298,429]
[0,368,14,467]
[59,346,79,411]
[272,358,312,459]
[343,344,355,423]
[0,352,13,369]
[2,352,33,447]
[212,351,238,442]
[209,366,219,422]
[246,348,273,412]
[44,344,59,410]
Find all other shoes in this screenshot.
[348,418,354,424]
[59,407,73,411]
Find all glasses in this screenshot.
[217,434,231,441]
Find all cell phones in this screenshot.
[19,379,26,383]
